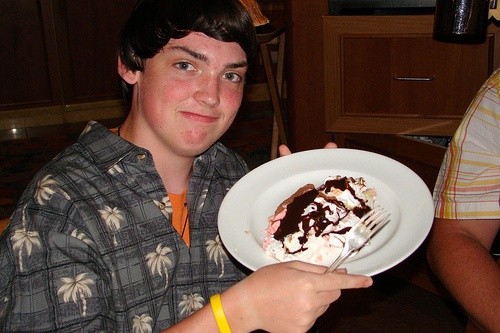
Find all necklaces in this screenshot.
[114,123,189,239]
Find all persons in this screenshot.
[423,66,500,333]
[0,0,374,333]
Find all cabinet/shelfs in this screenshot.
[0,0,288,135]
[321,13,500,305]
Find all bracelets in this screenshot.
[208,292,233,333]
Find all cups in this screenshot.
[432,0,491,45]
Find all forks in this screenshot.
[326,205,392,272]
[217,147,435,278]
